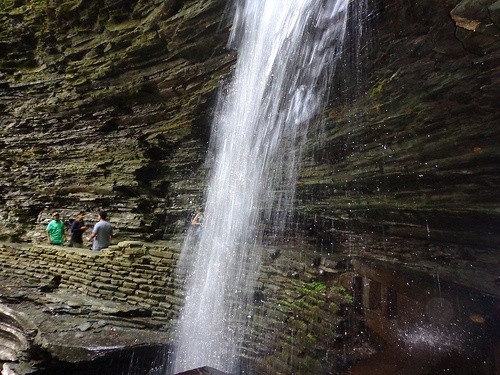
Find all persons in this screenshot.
[69,211,88,248]
[87,211,114,250]
[191,209,204,245]
[46,211,68,247]
